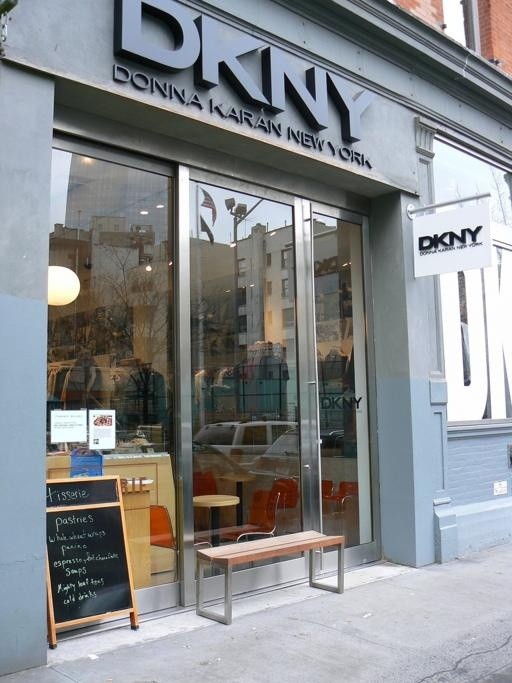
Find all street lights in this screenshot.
[224,197,267,366]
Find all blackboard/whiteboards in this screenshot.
[46,475,136,629]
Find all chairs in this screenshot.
[192,472,219,530]
[272,477,299,531]
[324,481,358,519]
[218,490,281,541]
[322,479,334,520]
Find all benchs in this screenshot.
[195,530,346,625]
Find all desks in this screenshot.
[193,495,240,547]
[215,474,257,526]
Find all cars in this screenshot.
[191,421,344,514]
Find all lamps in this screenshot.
[47,265,81,306]
